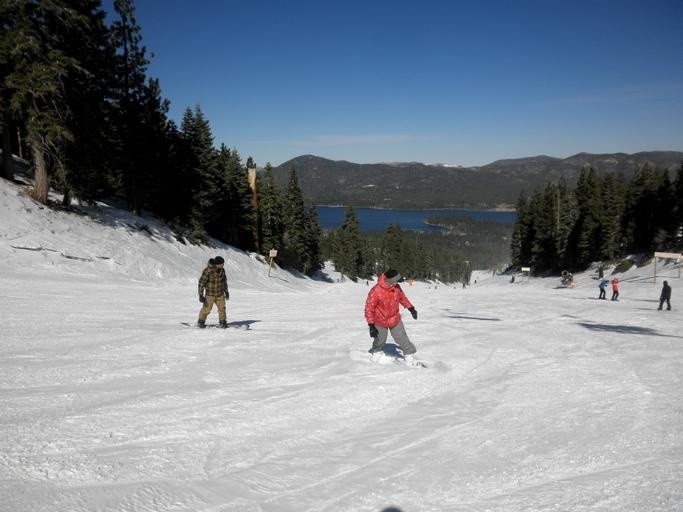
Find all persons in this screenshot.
[656,280,671,310]
[609,277,618,300]
[197,256,229,330]
[363,269,418,357]
[597,279,608,300]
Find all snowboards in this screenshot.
[181,323,249,331]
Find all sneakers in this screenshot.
[370,351,385,362]
[404,354,427,367]
[197,319,205,328]
[218,320,228,328]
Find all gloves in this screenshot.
[224,292,229,301]
[368,323,379,338]
[407,306,417,320]
[198,295,206,304]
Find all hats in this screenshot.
[384,270,402,284]
[213,256,224,265]
